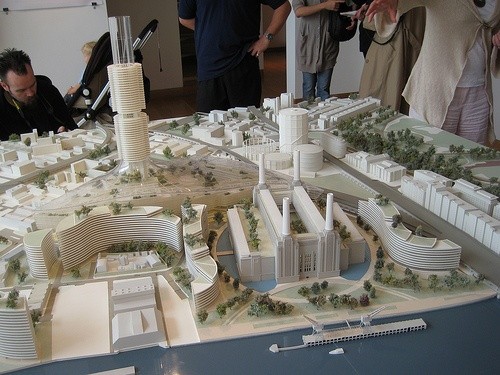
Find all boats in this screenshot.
[329,348,345,355]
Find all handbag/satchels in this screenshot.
[327,0,358,41]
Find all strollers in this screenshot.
[67,19,159,128]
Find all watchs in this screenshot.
[263,31,274,41]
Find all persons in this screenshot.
[355,0,500,146]
[179,0,292,115]
[294,0,355,100]
[0,48,79,141]
[64,41,97,102]
